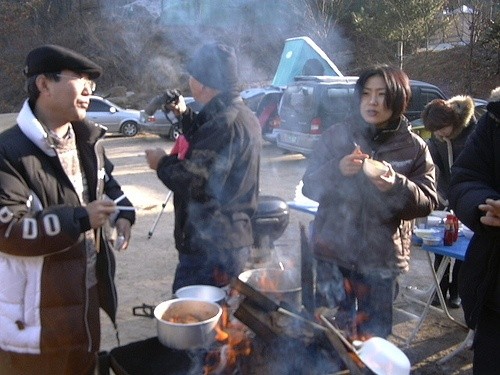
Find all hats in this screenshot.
[24,44,103,79]
[187,43,239,90]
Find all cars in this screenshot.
[84,96,143,136]
[254,90,285,141]
[139,93,196,139]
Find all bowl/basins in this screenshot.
[430,210,450,223]
[176,284,226,308]
[427,216,442,227]
[413,229,436,238]
[459,221,475,239]
[363,158,389,180]
[421,236,442,246]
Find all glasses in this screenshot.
[55,72,97,92]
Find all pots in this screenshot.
[237,267,303,315]
[152,298,223,349]
[319,314,411,375]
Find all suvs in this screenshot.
[273,75,451,156]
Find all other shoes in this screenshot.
[432,294,446,305]
[448,292,462,306]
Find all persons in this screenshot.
[301,65,438,342]
[0,45,136,375]
[144,41,262,299]
[447,87,500,375]
[425,95,478,308]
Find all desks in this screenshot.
[287,201,473,350]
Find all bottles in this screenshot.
[444,214,459,246]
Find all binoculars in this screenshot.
[145,88,182,117]
